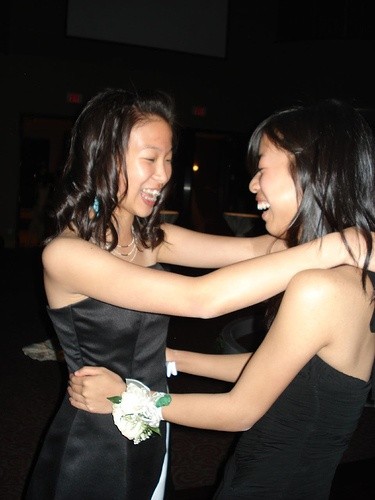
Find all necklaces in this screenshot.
[93,225,139,263]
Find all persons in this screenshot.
[21,86,375,500]
[65,97,375,500]
